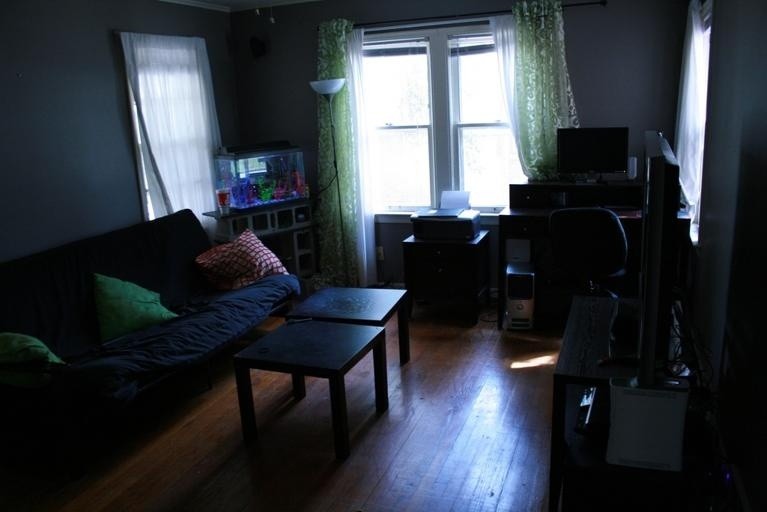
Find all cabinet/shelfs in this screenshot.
[400,228,492,326]
[546,288,718,512]
[201,195,323,305]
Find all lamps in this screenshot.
[309,76,353,288]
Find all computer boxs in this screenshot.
[505,262,536,331]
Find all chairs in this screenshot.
[545,203,628,323]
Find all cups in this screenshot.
[215,188,231,216]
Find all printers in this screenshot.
[410,209,481,240]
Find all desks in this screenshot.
[494,179,691,357]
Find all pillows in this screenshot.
[91,269,183,344]
[192,224,291,291]
[0,331,66,390]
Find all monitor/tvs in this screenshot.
[639,130,695,370]
[557,127,628,183]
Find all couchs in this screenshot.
[0,208,303,510]
